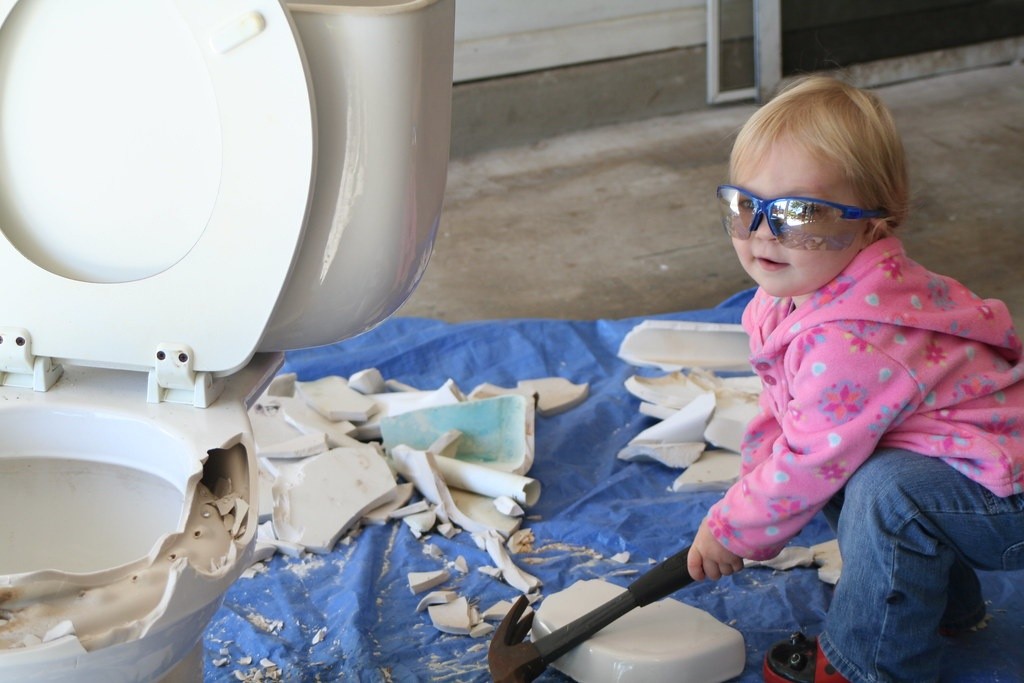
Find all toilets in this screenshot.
[2,2,453,683]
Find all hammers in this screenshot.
[488,544,696,683]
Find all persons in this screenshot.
[686,75,1024,683]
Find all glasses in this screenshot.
[715,184,896,252]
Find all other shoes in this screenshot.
[764,631,852,683]
